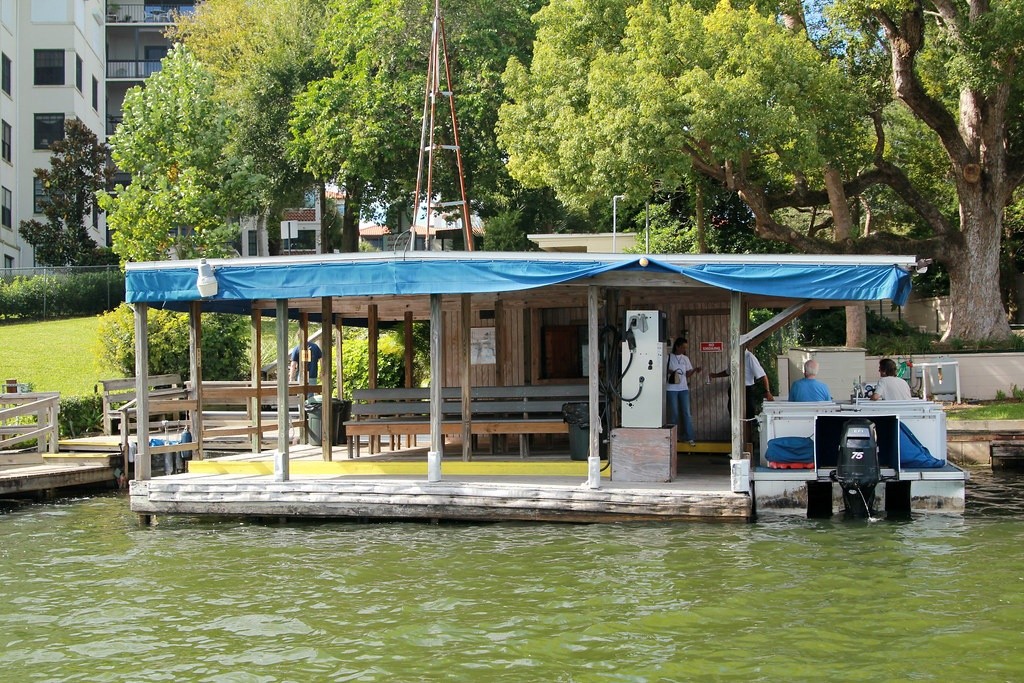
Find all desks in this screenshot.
[151,10,165,22]
[99,374,187,436]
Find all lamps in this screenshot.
[196,258,222,297]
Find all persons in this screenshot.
[289,332,322,412]
[788,360,831,402]
[666,338,701,447]
[709,349,774,466]
[870,359,912,400]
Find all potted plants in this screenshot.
[107,3,121,17]
[124,13,132,22]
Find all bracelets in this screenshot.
[764,390,769,392]
[290,377,295,379]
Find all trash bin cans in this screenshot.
[565,401,607,461]
[304,395,353,447]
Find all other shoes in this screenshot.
[688,440,696,446]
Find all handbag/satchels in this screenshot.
[668,368,683,384]
[765,436,814,463]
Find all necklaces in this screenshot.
[675,354,682,364]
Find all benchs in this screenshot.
[343,384,603,459]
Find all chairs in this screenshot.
[143,11,153,22]
[161,9,172,22]
[759,398,947,467]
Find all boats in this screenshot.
[751,376,971,515]
